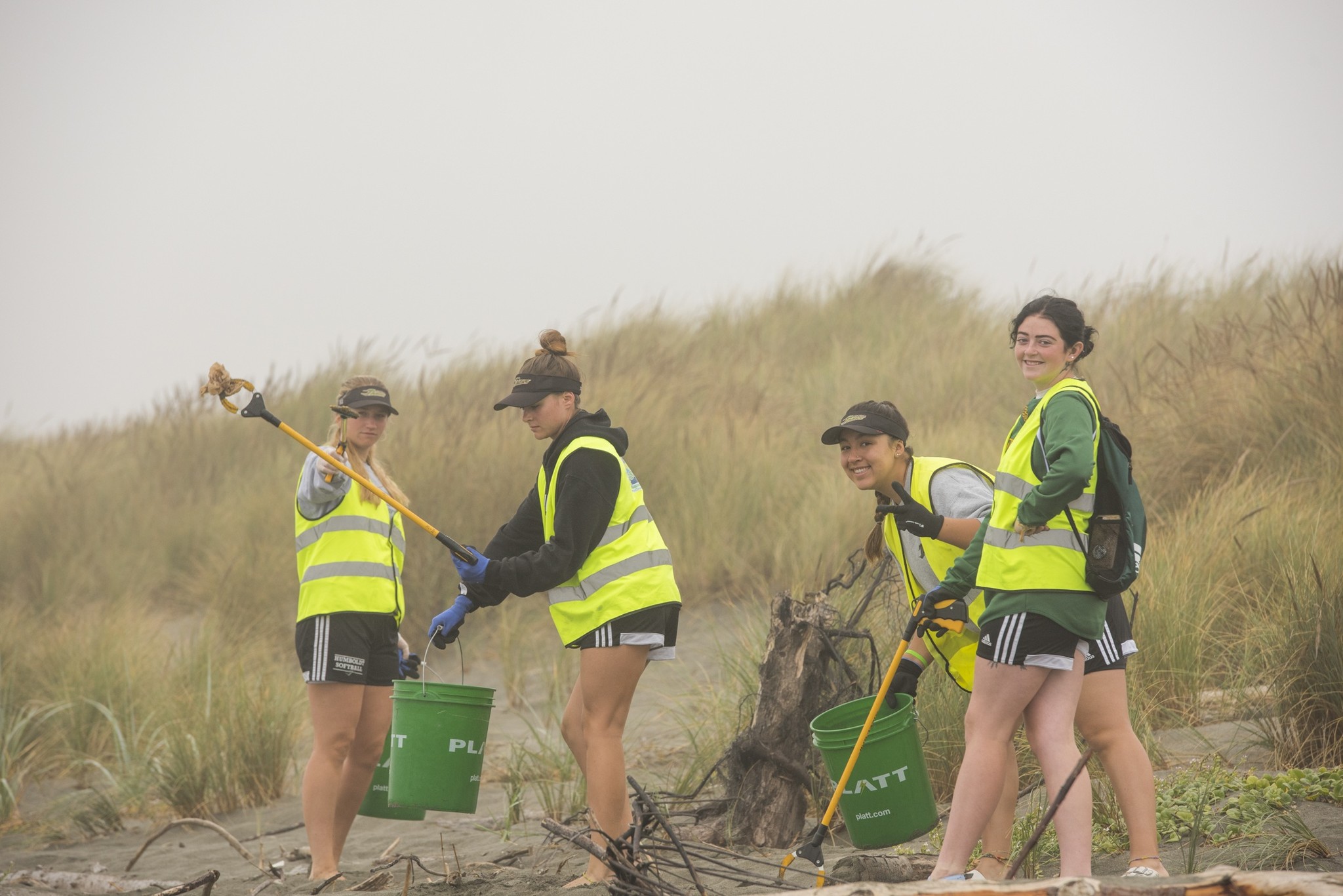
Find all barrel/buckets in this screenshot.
[809,692,941,850]
[386,624,496,814]
[355,659,445,820]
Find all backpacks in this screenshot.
[1039,385,1146,602]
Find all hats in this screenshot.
[339,386,399,416]
[821,411,907,445]
[493,373,582,411]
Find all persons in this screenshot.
[294,376,410,884]
[427,327,682,890]
[833,401,1171,881]
[922,294,1116,884]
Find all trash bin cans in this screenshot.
[810,693,939,850]
[357,661,444,821]
[388,625,496,814]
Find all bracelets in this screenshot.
[1017,515,1037,543]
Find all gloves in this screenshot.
[398,649,422,680]
[916,582,966,639]
[875,481,944,540]
[429,594,478,650]
[884,658,923,709]
[451,545,490,584]
